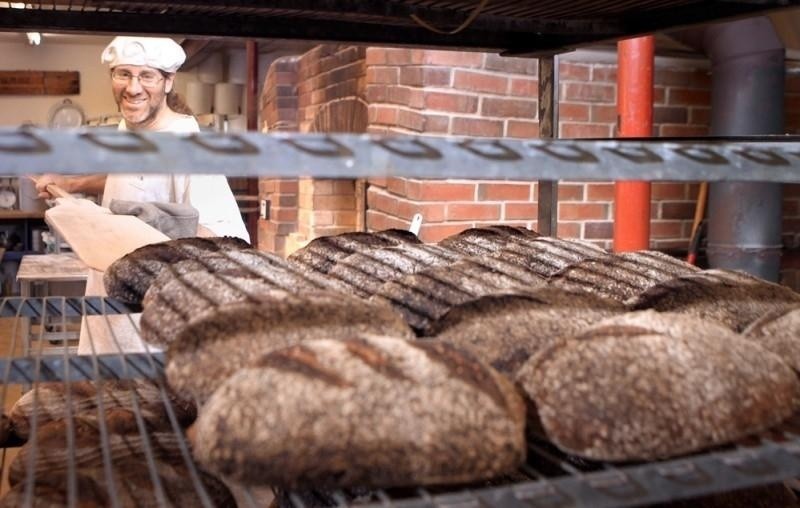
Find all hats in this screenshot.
[101,35,187,74]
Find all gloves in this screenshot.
[109,198,199,240]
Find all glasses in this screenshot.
[110,70,166,87]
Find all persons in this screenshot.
[33,36,254,356]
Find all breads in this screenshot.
[1,227,800,508]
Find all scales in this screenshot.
[0,176,20,211]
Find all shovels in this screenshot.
[25,173,173,273]
